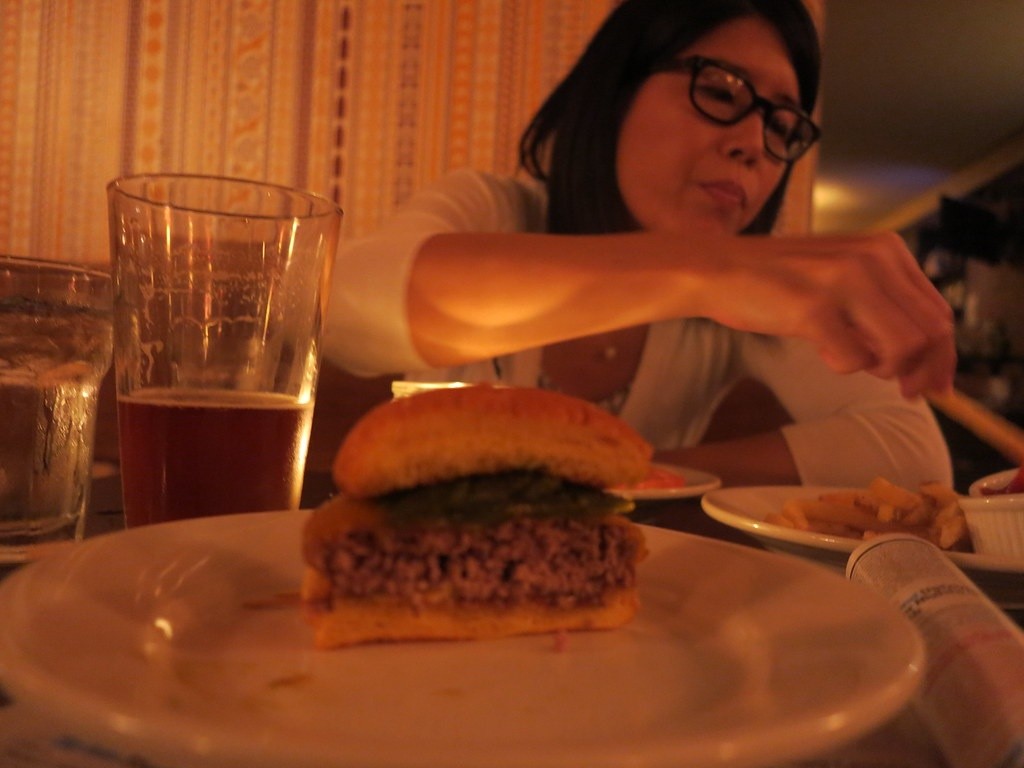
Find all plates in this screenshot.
[1,508,927,768]
[603,460,723,502]
[701,485,1024,588]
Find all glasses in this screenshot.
[652,54,824,163]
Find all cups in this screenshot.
[106,172,344,530]
[0,251,116,566]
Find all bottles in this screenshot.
[845,532,1024,768]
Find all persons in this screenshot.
[273,0,959,503]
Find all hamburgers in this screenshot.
[295,384,656,650]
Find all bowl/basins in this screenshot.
[959,493,1024,561]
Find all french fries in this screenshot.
[764,475,974,552]
[930,391,1024,463]
